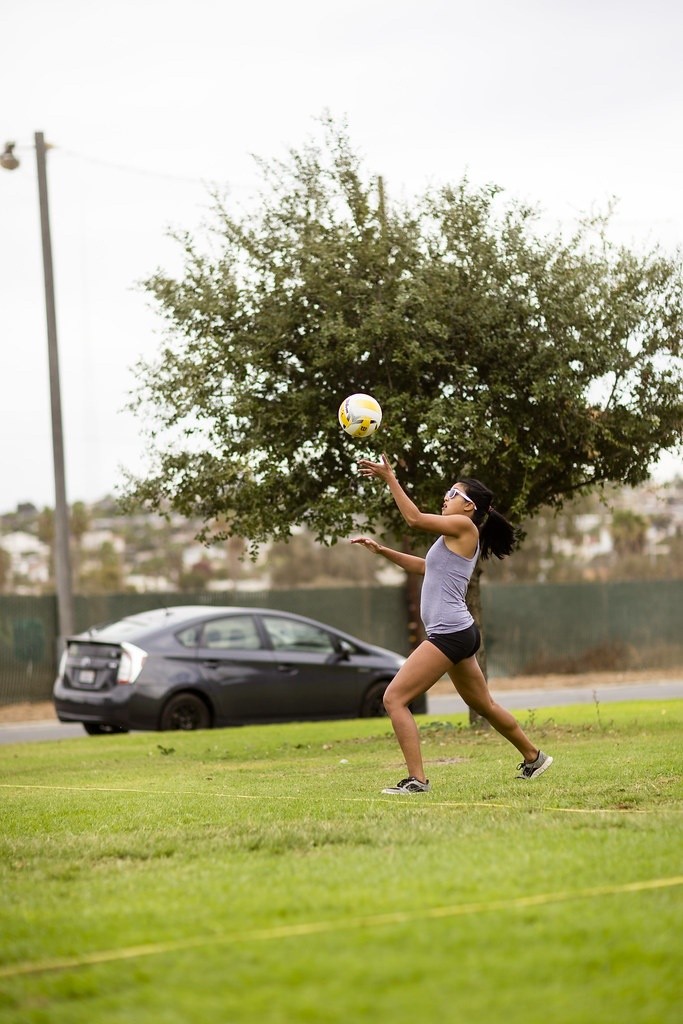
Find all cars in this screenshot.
[52,604,427,735]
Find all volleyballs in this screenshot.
[338,393,384,438]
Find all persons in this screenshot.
[350,454,553,794]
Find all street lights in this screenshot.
[0,130,77,658]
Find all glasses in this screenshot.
[444,486,479,513]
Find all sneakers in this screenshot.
[513,750,554,783]
[381,777,430,797]
